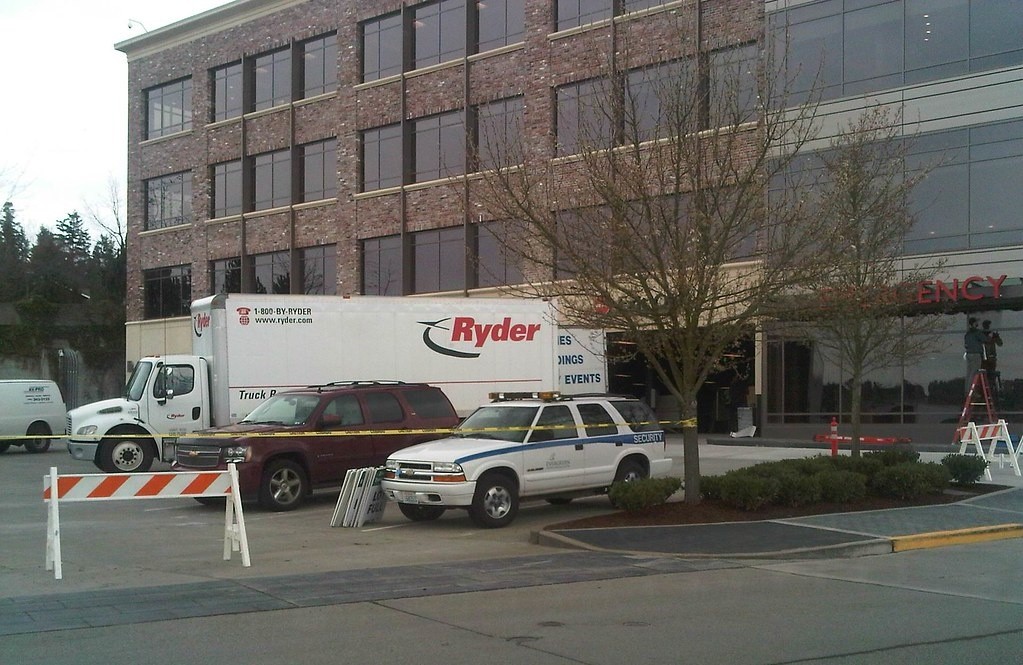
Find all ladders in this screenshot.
[951,369,997,445]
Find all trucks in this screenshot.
[0,379,67,454]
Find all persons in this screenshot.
[980,319,1003,389]
[730,376,750,433]
[964,317,994,398]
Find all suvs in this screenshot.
[174,377,460,515]
[382,391,677,528]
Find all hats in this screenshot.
[968,317,976,325]
[983,320,991,326]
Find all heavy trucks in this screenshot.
[66,292,557,472]
